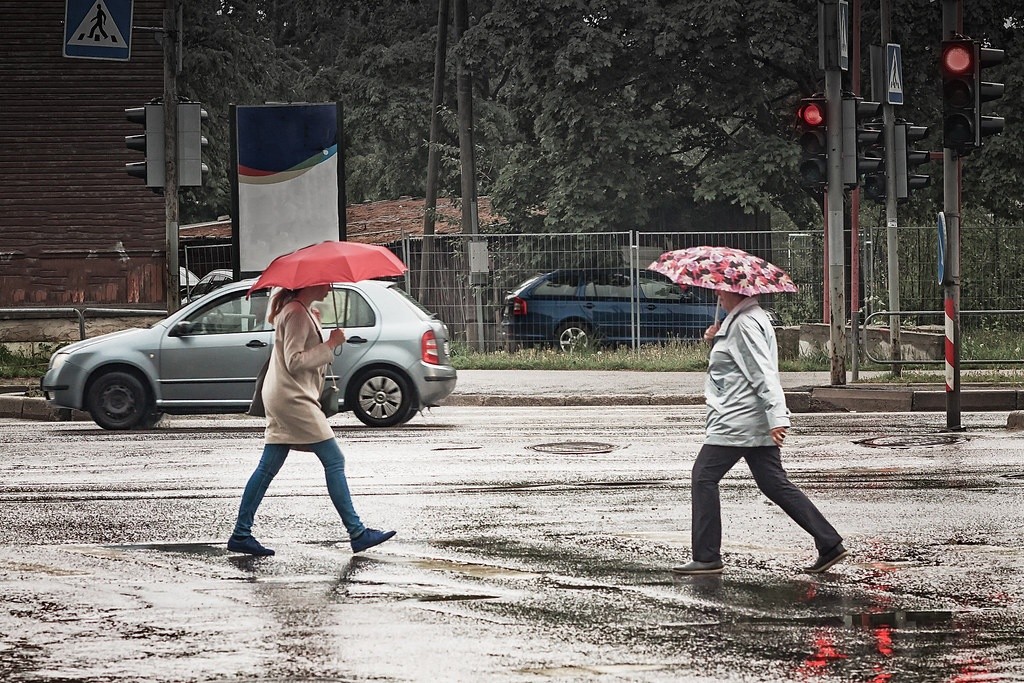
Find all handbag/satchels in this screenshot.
[321,386,339,418]
[244,356,271,418]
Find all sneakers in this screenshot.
[351,528,397,553]
[226,534,275,555]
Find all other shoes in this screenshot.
[671,558,724,574]
[805,542,849,572]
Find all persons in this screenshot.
[671,290,849,574]
[226,284,397,557]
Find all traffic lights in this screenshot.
[979,46,1006,146]
[893,118,932,198]
[124,97,166,193]
[938,38,982,147]
[841,91,883,191]
[177,101,209,187]
[794,92,829,187]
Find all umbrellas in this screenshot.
[645,246,800,334]
[245,237,409,356]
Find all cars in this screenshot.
[500,267,783,356]
[40,264,457,431]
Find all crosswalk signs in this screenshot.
[887,43,904,105]
[837,0,849,72]
[62,1,133,61]
